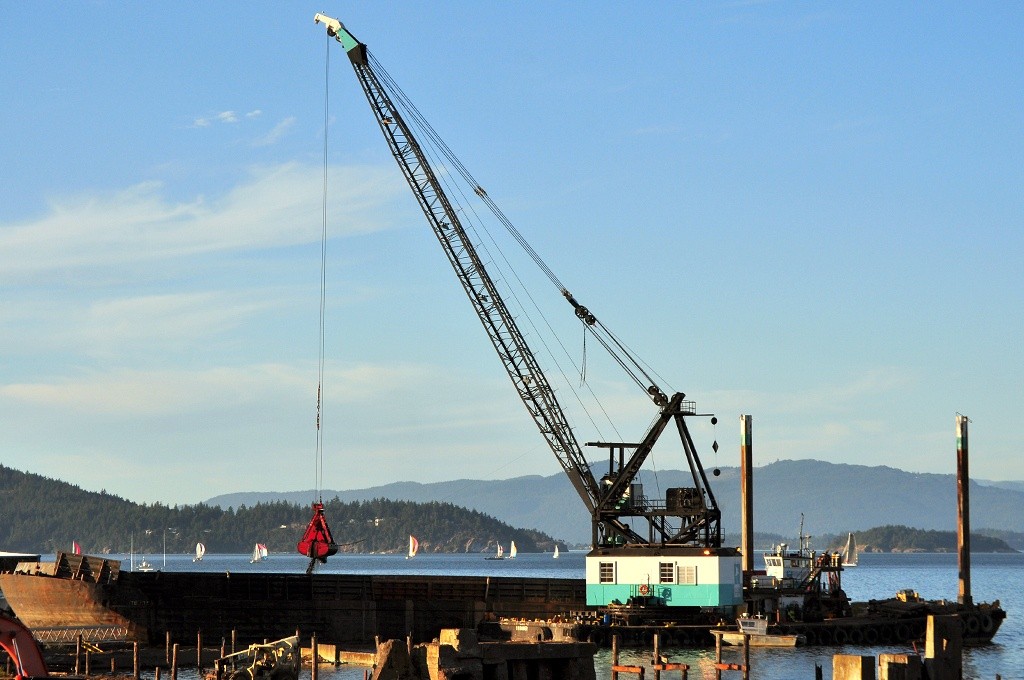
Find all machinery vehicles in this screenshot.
[297,9,743,609]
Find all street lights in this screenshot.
[164,527,172,568]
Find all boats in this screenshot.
[479,410,1008,650]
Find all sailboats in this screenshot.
[192,542,205,563]
[485,539,518,560]
[837,532,859,567]
[249,542,268,564]
[405,535,420,560]
[553,545,559,558]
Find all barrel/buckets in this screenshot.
[604,614,610,624]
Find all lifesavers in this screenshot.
[640,585,649,595]
